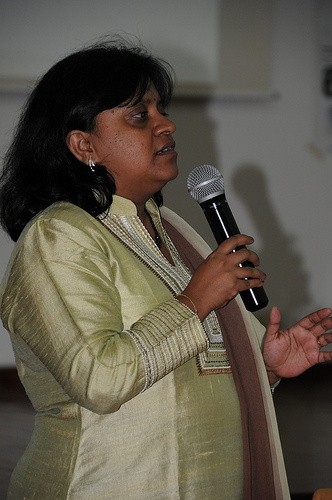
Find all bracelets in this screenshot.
[176,293,199,316]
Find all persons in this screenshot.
[0,33,332,500]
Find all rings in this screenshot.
[317,336,325,348]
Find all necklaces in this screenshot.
[143,206,163,250]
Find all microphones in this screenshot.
[186,164,270,311]
